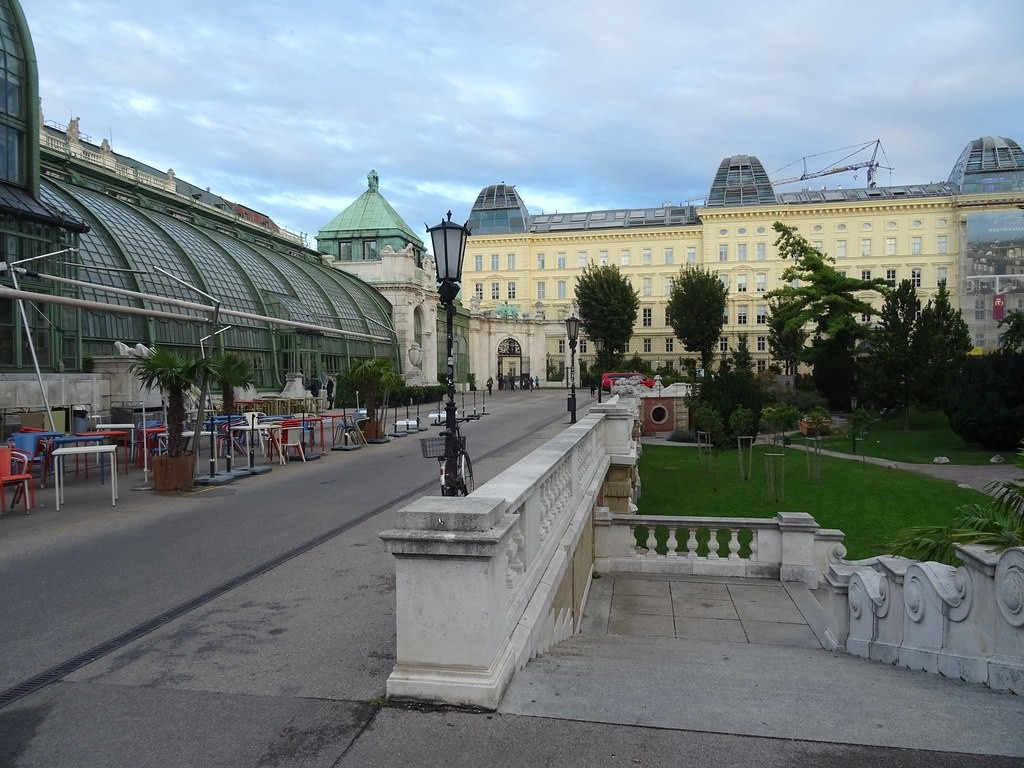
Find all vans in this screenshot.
[602,372,657,393]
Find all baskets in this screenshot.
[420,437,456,459]
[458,436,467,450]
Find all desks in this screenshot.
[319,413,345,448]
[296,418,327,451]
[158,431,218,473]
[54,435,106,487]
[52,445,117,512]
[75,430,128,479]
[95,424,134,465]
[229,425,283,467]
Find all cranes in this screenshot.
[685,138,894,202]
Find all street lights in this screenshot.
[594,338,605,404]
[426,212,471,494]
[563,312,581,424]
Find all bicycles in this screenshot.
[420,413,481,497]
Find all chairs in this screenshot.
[206,412,318,465]
[133,420,168,469]
[335,413,370,448]
[0,426,64,517]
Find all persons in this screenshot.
[498,374,504,390]
[486,377,493,396]
[326,375,334,410]
[535,376,540,389]
[589,377,596,398]
[530,377,533,391]
[510,376,515,391]
[524,376,529,390]
[308,375,322,397]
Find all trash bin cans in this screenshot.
[72,410,88,433]
